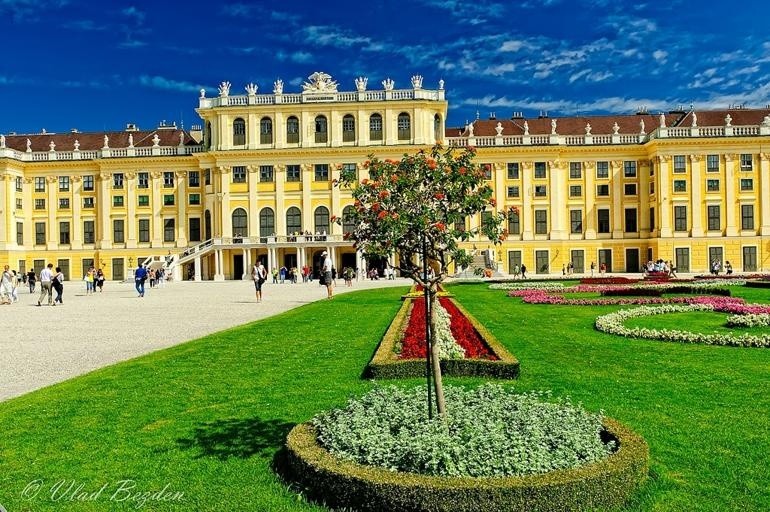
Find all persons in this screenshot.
[590,262,598,277]
[562,261,575,275]
[319,251,333,298]
[270,265,397,289]
[52,267,65,305]
[234,232,243,243]
[251,260,267,303]
[263,229,327,242]
[513,264,521,279]
[135,264,148,297]
[642,258,679,278]
[711,257,733,277]
[0,260,39,306]
[599,262,607,277]
[520,264,527,279]
[143,267,173,288]
[84,265,105,295]
[38,263,56,306]
[483,264,493,278]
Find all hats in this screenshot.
[320,251,327,257]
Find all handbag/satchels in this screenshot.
[258,278,264,286]
[319,275,327,286]
[52,277,63,292]
